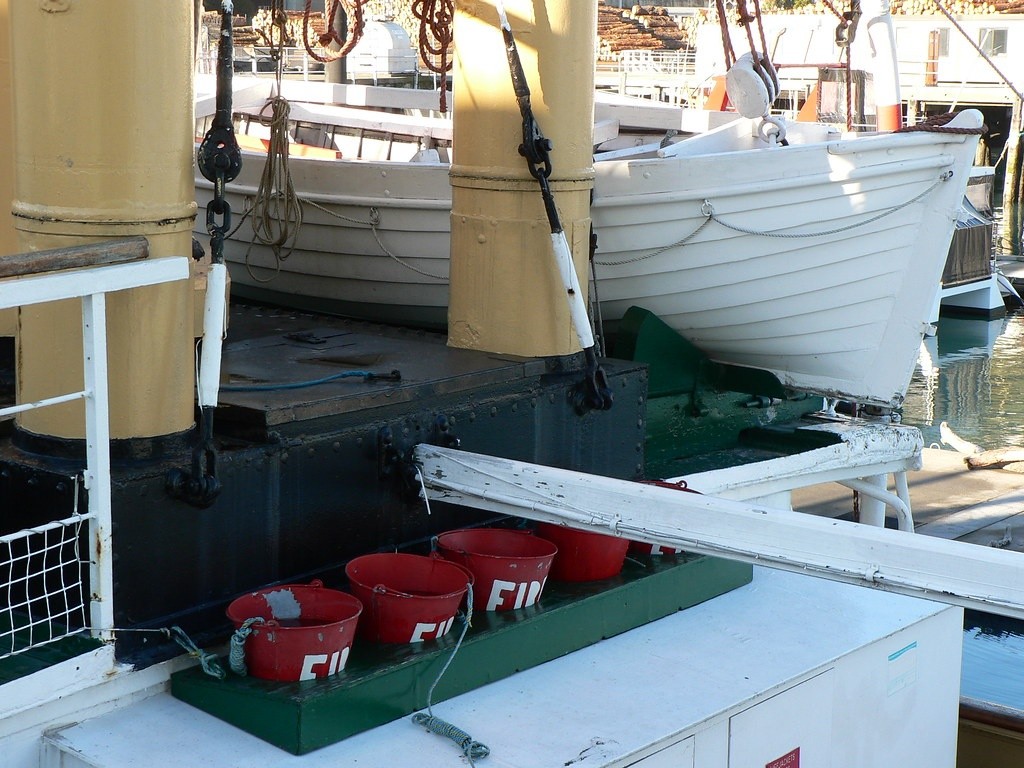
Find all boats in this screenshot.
[193,66,983,415]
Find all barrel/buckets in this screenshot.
[433,527,559,611]
[224,580,364,683]
[345,551,474,645]
[633,478,704,556]
[537,518,631,582]
